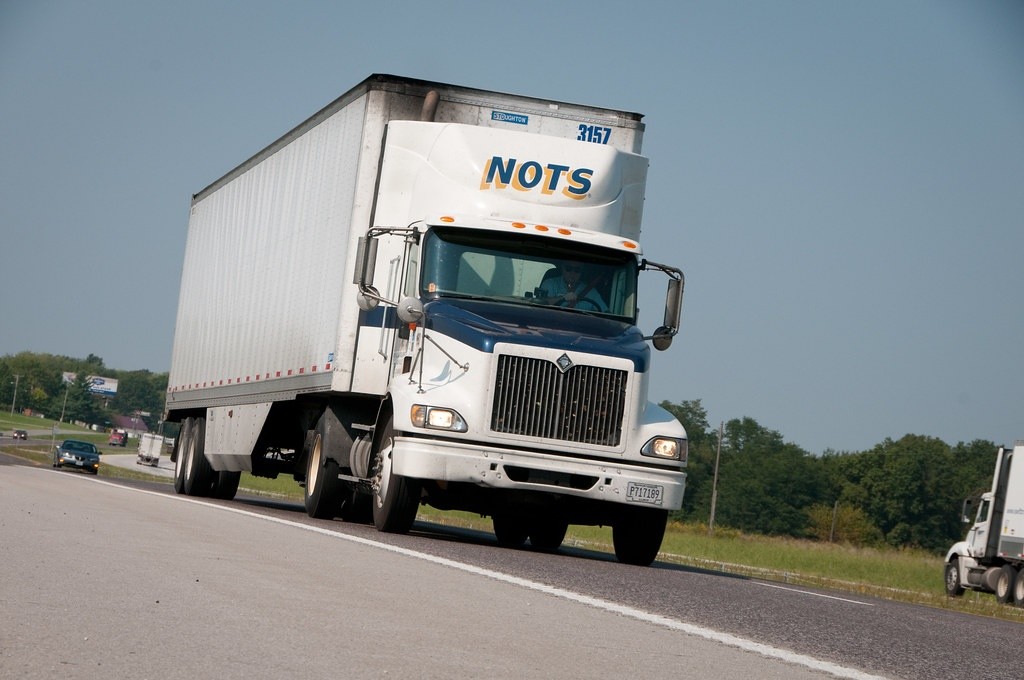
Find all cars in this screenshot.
[52,440,104,476]
[108,429,128,447]
[12,430,28,440]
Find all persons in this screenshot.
[537,252,612,314]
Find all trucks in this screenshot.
[137,432,164,467]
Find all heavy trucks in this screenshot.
[944,441,1024,609]
[157,71,691,566]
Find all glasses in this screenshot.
[565,267,581,273]
